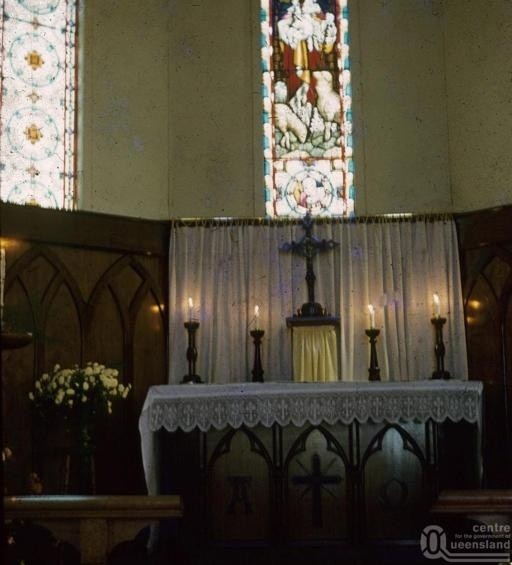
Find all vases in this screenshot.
[61,449,97,495]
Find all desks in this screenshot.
[0,495,185,565]
[430,489,512,564]
[138,379,483,565]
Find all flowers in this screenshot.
[27,360,132,462]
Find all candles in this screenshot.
[434,294,440,315]
[189,298,194,320]
[254,305,260,330]
[368,304,375,330]
